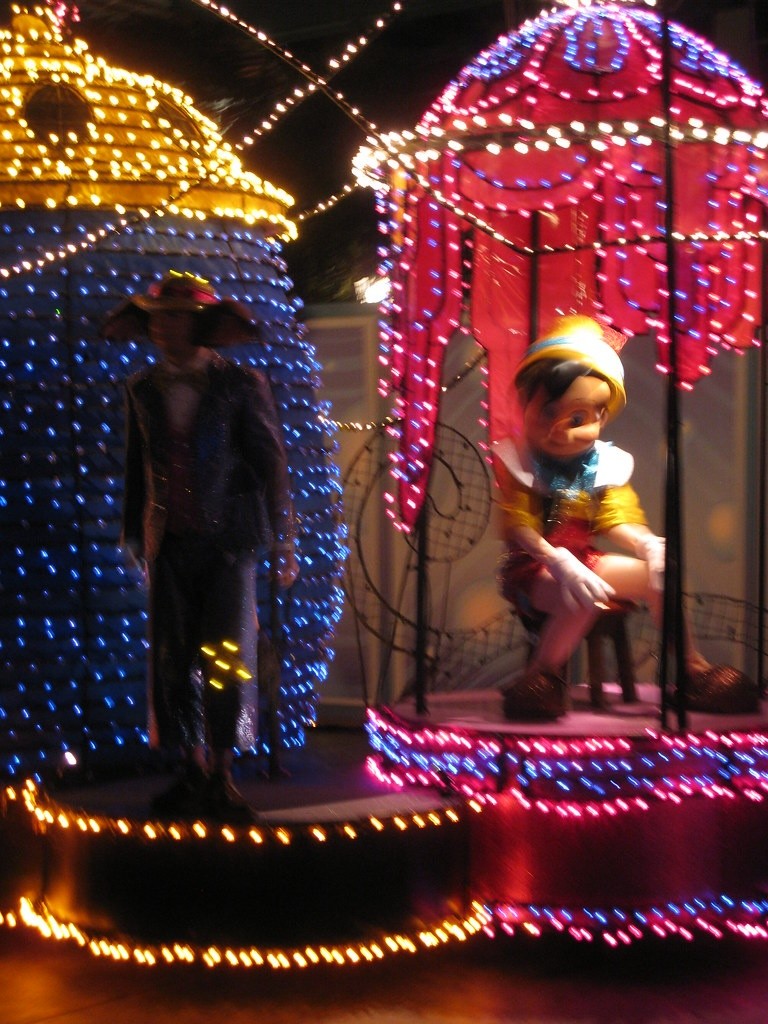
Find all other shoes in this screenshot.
[204,770,264,823]
[150,773,209,812]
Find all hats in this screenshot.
[102,277,264,349]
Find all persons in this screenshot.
[489,314,759,721]
[98,271,299,824]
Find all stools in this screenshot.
[513,602,636,712]
